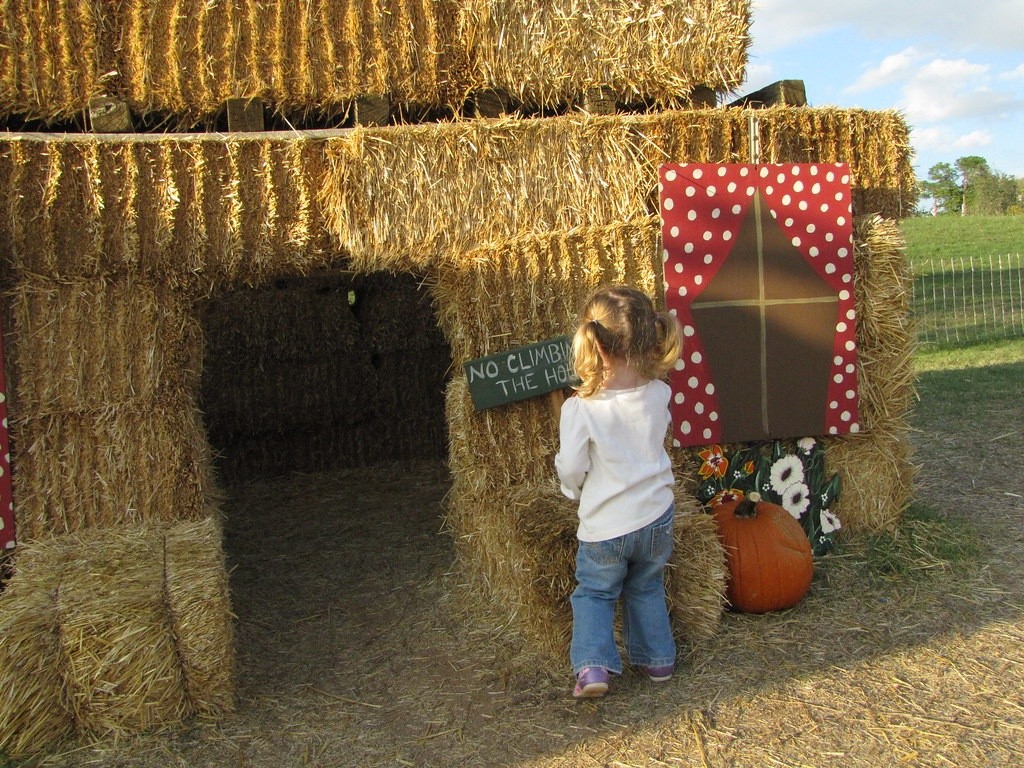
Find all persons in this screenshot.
[554,284,680,700]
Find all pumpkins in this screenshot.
[708,494,814,614]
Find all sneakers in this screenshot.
[572,666,608,698]
[644,664,673,682]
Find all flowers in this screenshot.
[696,434,845,556]
